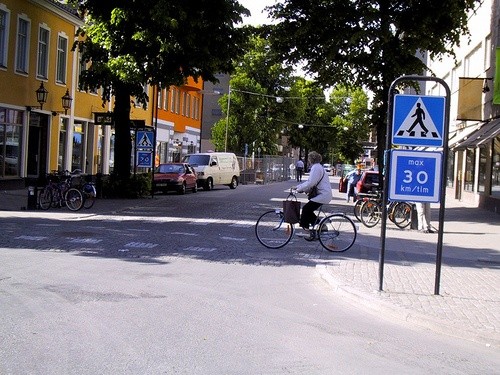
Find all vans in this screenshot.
[180,152,241,192]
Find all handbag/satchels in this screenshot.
[282,194,301,223]
[305,185,319,200]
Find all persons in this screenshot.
[343,169,362,202]
[296,158,304,182]
[291,151,332,238]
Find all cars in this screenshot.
[339,169,379,203]
[272,163,284,175]
[323,164,334,172]
[155,163,197,195]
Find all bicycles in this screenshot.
[354,183,413,228]
[255,188,357,253]
[38,170,96,211]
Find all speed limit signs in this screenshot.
[388,150,442,203]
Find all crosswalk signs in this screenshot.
[391,94,446,147]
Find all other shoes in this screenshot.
[297,180,302,182]
[313,225,328,231]
[295,229,310,237]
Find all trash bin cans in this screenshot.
[411,202,418,229]
